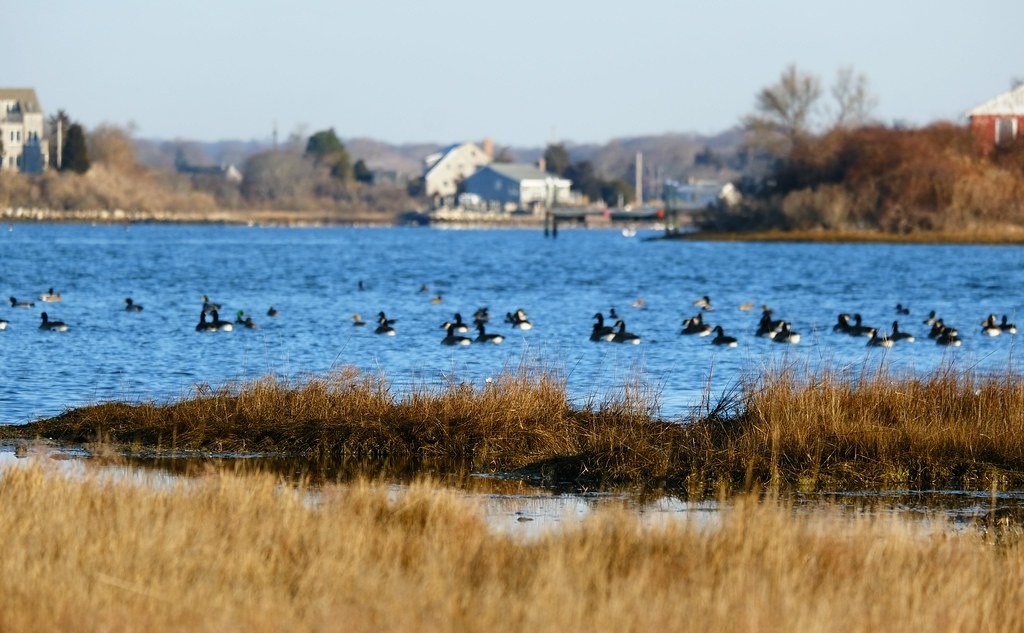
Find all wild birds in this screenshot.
[39,312,68,332]
[38,288,61,302]
[352,280,533,348]
[695,295,713,312]
[590,298,645,345]
[832,304,1017,348]
[125,299,143,312]
[739,303,800,344]
[711,326,738,347]
[681,310,712,336]
[196,295,279,333]
[8,297,34,308]
